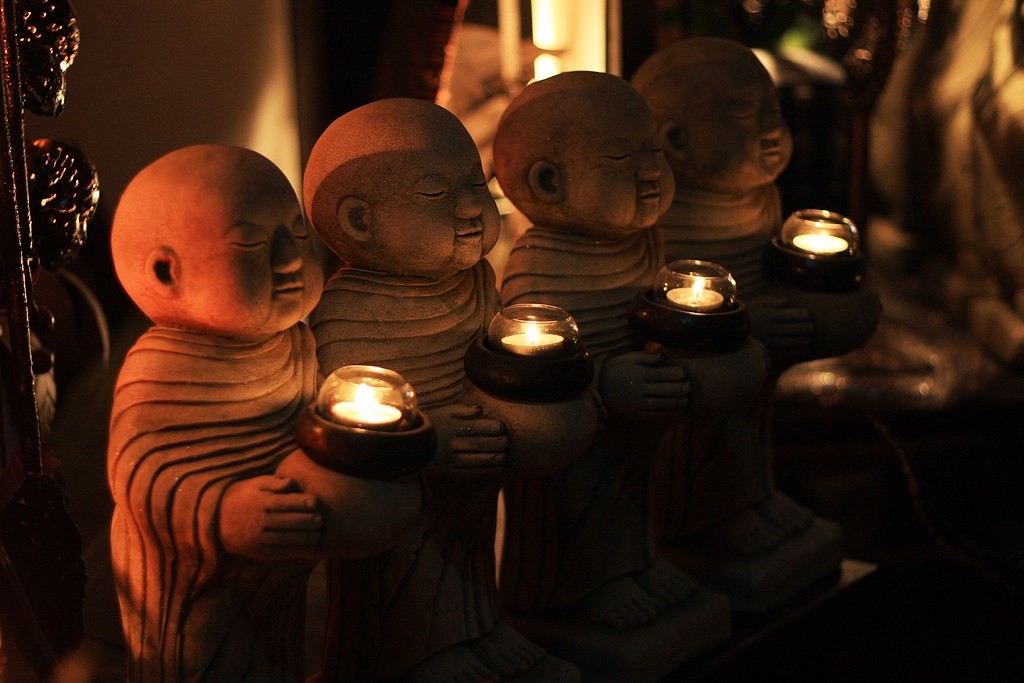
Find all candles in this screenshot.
[666,276,724,312]
[793,230,848,254]
[502,321,564,355]
[332,381,402,429]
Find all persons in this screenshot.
[110,142,327,683]
[301,37,819,683]
